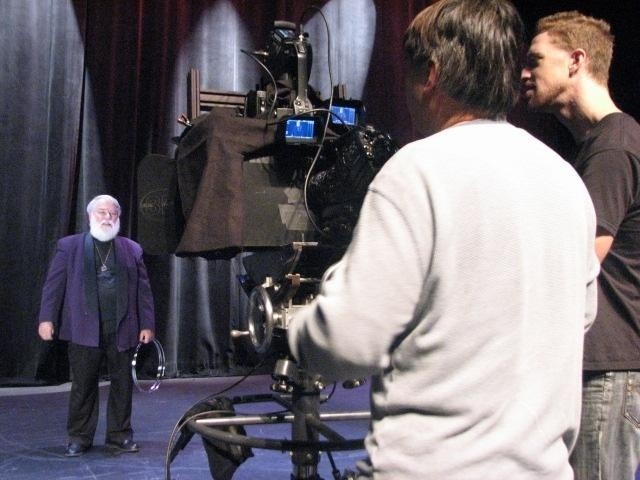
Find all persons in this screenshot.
[292,120,303,137]
[521,9,639,480]
[277,0,602,480]
[337,107,346,122]
[36,193,158,457]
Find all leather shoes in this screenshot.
[105,436,139,453]
[64,442,90,457]
